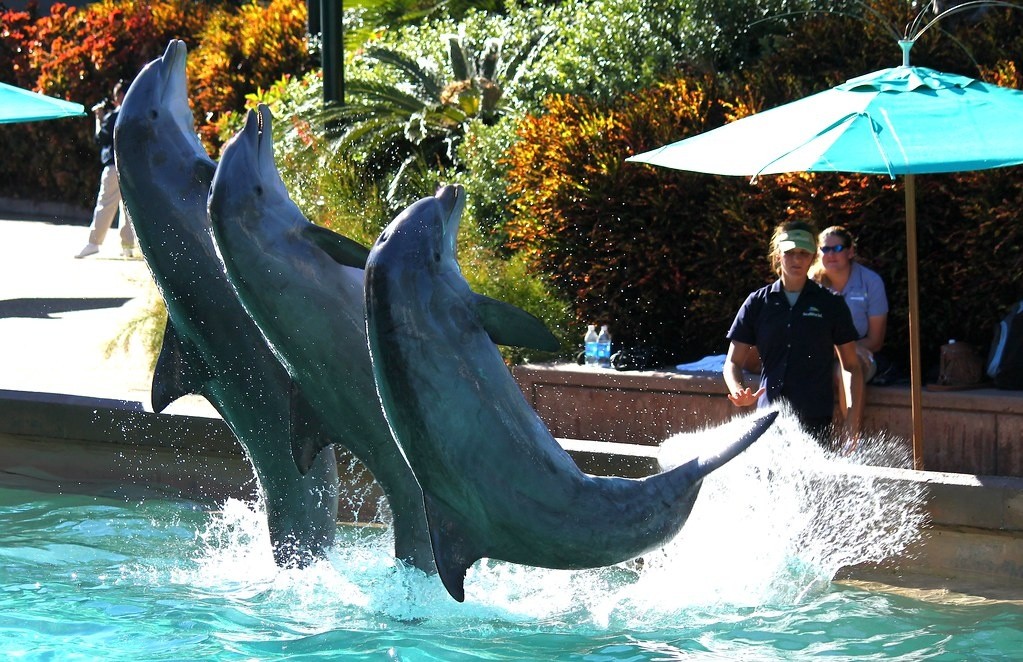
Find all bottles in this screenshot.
[597,326,611,368]
[940,339,965,385]
[584,325,598,367]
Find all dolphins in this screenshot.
[112,40,341,573]
[364,185,778,604]
[206,103,437,578]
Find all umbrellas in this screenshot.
[627,68,1023,473]
[1,79,89,123]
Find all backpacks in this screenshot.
[936,342,977,391]
[983,299,1023,390]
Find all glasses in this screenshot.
[821,245,849,253]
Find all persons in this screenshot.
[724,218,849,455]
[807,222,888,453]
[75,77,136,257]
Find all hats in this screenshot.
[774,230,817,253]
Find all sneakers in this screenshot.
[74,245,99,259]
[119,249,134,257]
